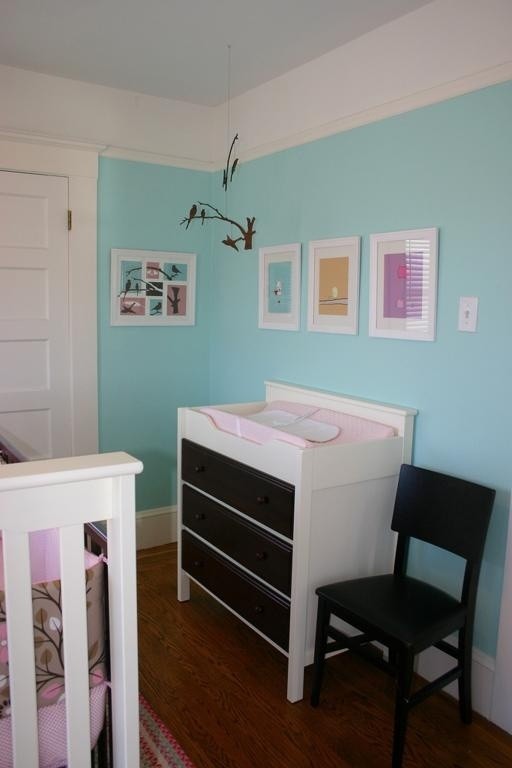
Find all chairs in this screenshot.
[309,463,497,765]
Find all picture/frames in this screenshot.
[306,236,360,335]
[110,249,196,327]
[369,228,438,340]
[257,243,301,332]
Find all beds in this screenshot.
[0,424,144,768]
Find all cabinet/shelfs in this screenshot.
[176,376,419,703]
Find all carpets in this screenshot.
[138,687,200,768]
